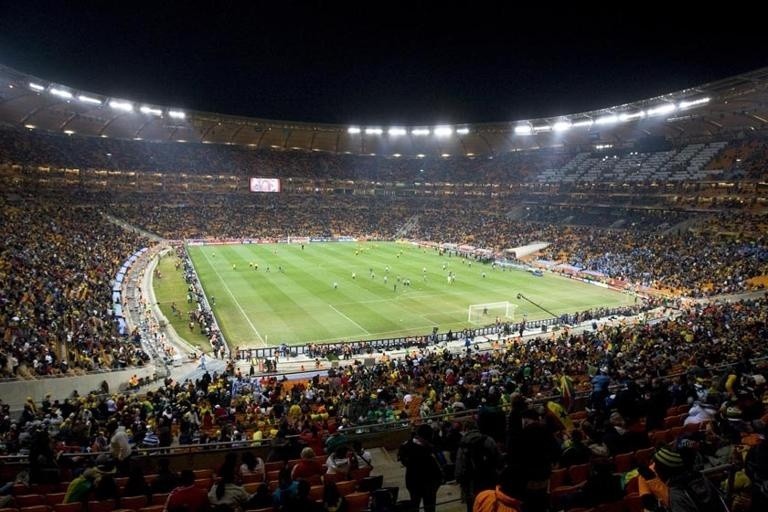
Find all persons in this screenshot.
[1,124,214,334]
[438,135,768,327]
[1,327,767,512]
[216,134,439,290]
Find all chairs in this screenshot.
[1,399,767,512]
